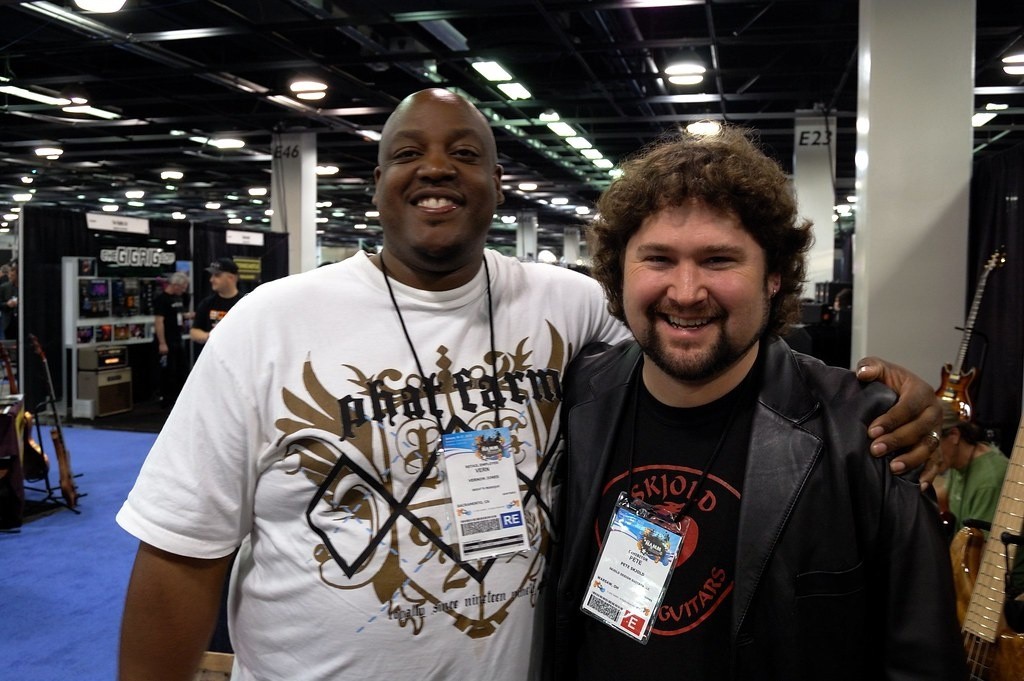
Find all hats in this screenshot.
[938,399,961,429]
[203,258,239,273]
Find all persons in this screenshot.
[152,271,188,403]
[189,257,249,366]
[0,258,19,341]
[527,128,970,681]
[934,396,1009,543]
[116,89,945,681]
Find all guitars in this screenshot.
[933,243,1006,422]
[0,341,50,479]
[26,332,79,507]
[945,413,1024,681]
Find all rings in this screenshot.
[929,430,940,447]
